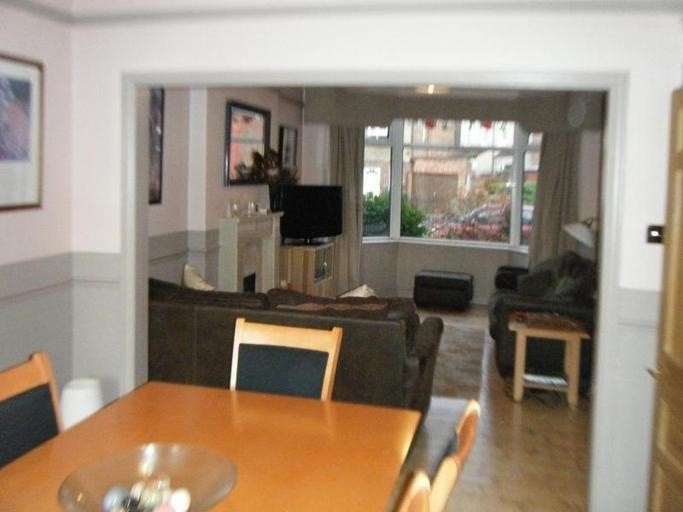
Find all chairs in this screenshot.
[431,397,479,511]
[395,468,431,512]
[230,316,343,401]
[0,351,65,467]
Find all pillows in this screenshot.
[148,277,267,309]
[340,283,377,297]
[544,264,594,306]
[269,288,388,319]
[181,263,215,291]
[518,268,551,296]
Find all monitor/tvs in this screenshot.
[278,185,343,246]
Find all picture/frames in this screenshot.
[279,126,298,170]
[150,87,165,204]
[226,99,272,184]
[0,54,46,209]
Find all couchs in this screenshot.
[147,302,445,434]
[489,258,596,377]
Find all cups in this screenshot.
[229,198,241,218]
[247,202,257,217]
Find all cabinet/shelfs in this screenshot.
[218,213,274,292]
[282,242,336,296]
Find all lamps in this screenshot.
[560,217,597,250]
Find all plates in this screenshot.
[58,443,236,512]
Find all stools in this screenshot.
[414,269,474,312]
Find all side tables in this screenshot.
[509,311,590,406]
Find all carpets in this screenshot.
[431,327,485,398]
